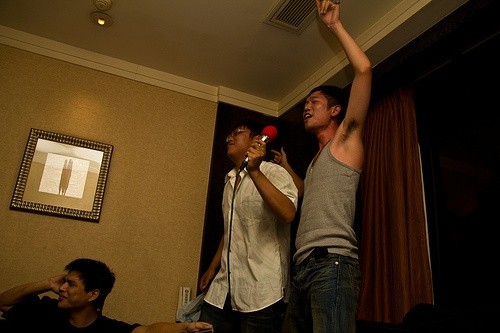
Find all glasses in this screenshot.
[226,128,257,143]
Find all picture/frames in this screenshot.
[9,128,114,223]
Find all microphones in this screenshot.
[240,125,277,171]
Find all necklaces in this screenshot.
[308,139,331,176]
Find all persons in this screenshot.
[270,0,373,332]
[0,258,214,333]
[197,120,299,333]
